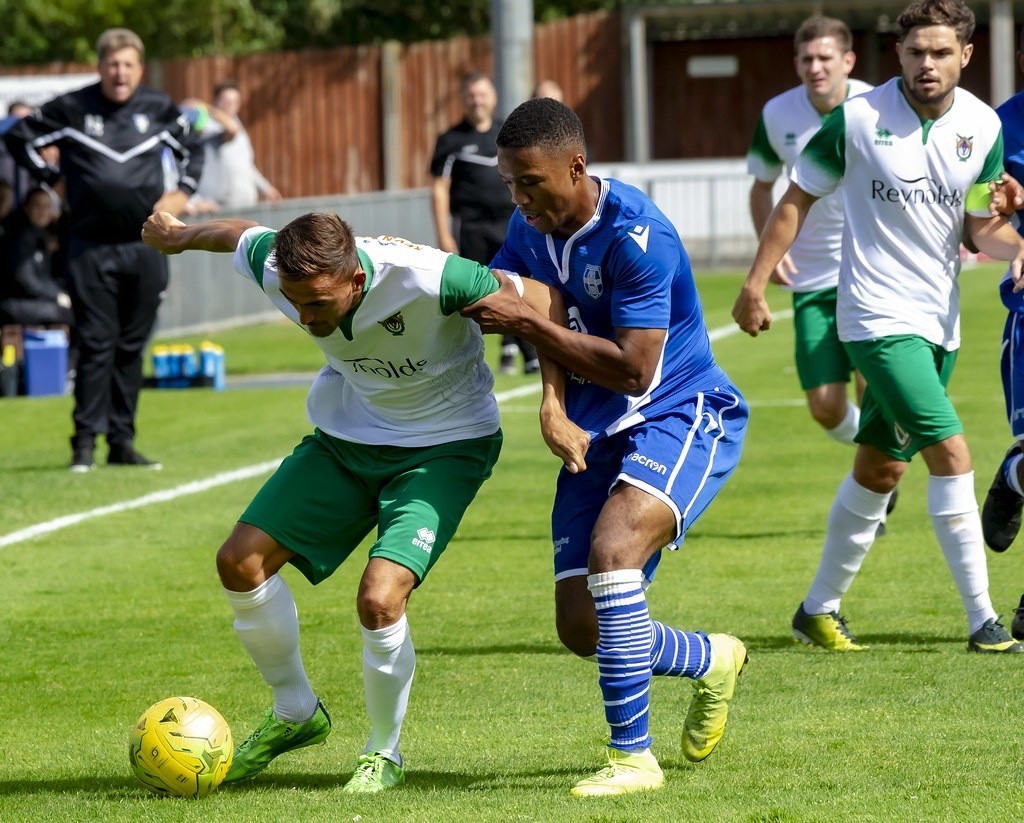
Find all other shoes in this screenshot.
[523,358,540,373]
[498,355,519,376]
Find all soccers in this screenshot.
[128,696,235,799]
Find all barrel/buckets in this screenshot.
[24,329,68,395]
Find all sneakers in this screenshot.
[681,632,750,762]
[222,698,331,784]
[570,748,666,798]
[982,440,1024,552]
[969,615,1024,652]
[107,445,161,472]
[70,447,98,472]
[876,490,898,537]
[342,753,404,794]
[791,602,871,651]
[1011,595,1024,640]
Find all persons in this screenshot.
[0,86,283,329]
[459,99,747,798]
[1,26,205,474]
[139,210,591,798]
[430,68,567,375]
[732,0,1024,652]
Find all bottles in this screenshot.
[0,345,17,394]
[151,341,224,393]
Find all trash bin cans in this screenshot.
[23,328,69,396]
